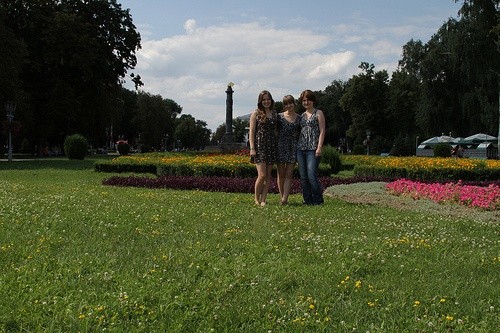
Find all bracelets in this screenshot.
[250,147,256,150]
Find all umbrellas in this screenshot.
[421,134,497,149]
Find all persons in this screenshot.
[297,90,326,205]
[275,95,302,205]
[248,91,278,207]
